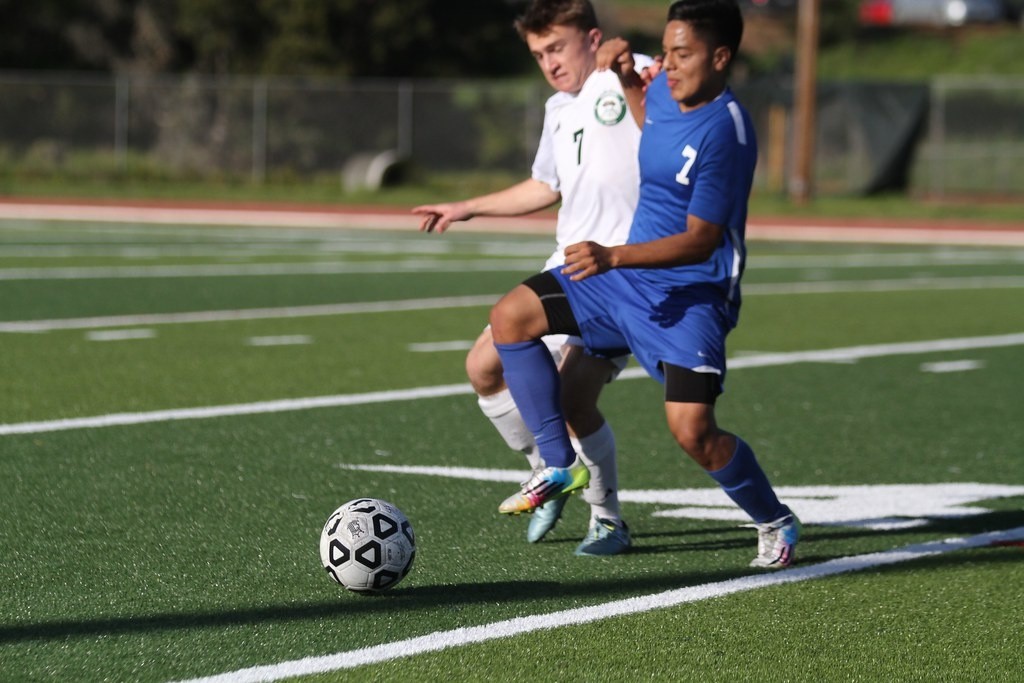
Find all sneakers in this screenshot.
[498,453,591,516]
[574,517,631,557]
[527,491,571,543]
[749,502,801,568]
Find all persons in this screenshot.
[487,0,802,570]
[411,0,665,555]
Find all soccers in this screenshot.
[319,497,416,594]
